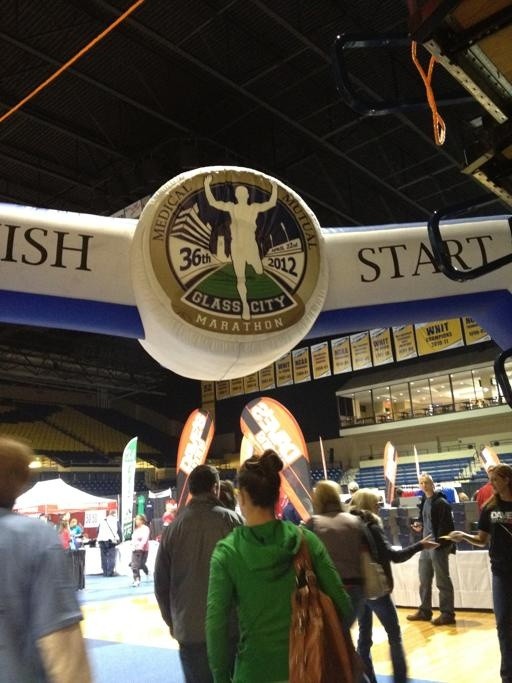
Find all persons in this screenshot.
[200,447,355,682]
[297,474,383,632]
[446,463,512,683]
[1,433,97,683]
[151,463,246,683]
[38,496,181,588]
[345,486,441,682]
[403,471,458,625]
[342,480,494,509]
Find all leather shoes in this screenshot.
[407,614,455,625]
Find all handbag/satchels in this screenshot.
[362,551,390,601]
[289,536,364,683]
